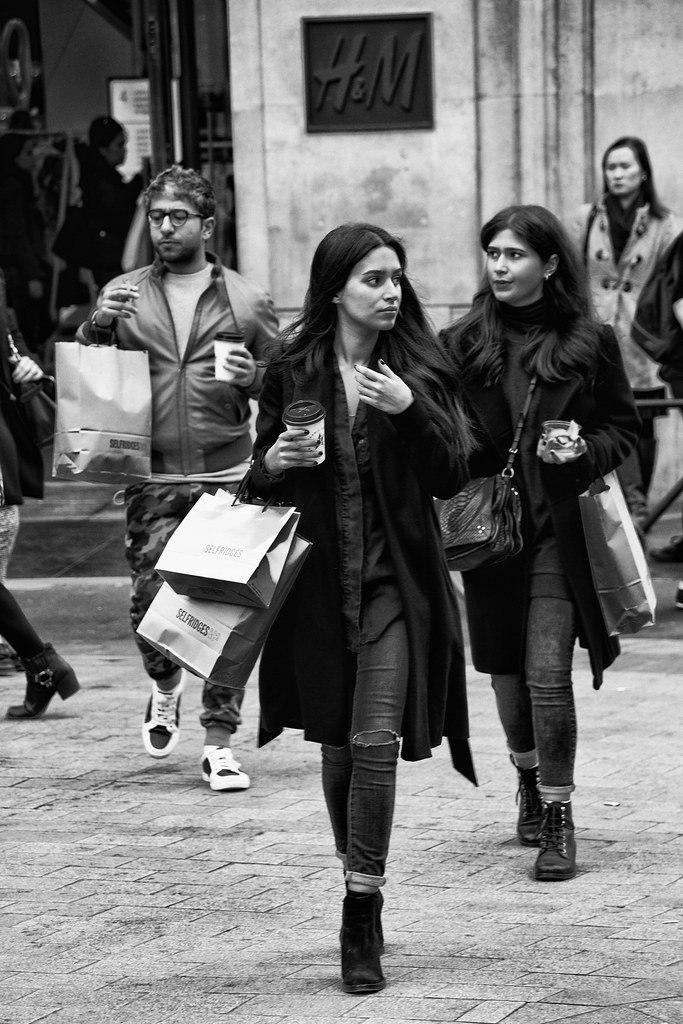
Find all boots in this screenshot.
[343,870,385,956]
[6,642,80,719]
[509,753,546,846]
[637,440,656,495]
[535,794,576,880]
[339,894,386,993]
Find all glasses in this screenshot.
[146,209,207,227]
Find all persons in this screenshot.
[0,109,152,364]
[439,205,642,879]
[564,138,683,608]
[77,167,280,790]
[0,280,80,720]
[252,224,479,993]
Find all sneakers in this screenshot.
[199,745,250,790]
[142,668,187,760]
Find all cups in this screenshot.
[542,420,583,458]
[281,399,326,467]
[213,331,248,382]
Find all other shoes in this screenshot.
[0,643,16,675]
[650,535,683,562]
[12,652,25,671]
[675,581,683,608]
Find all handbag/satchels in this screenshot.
[135,461,313,692]
[51,310,152,484]
[579,467,656,637]
[433,475,524,572]
[19,375,54,448]
